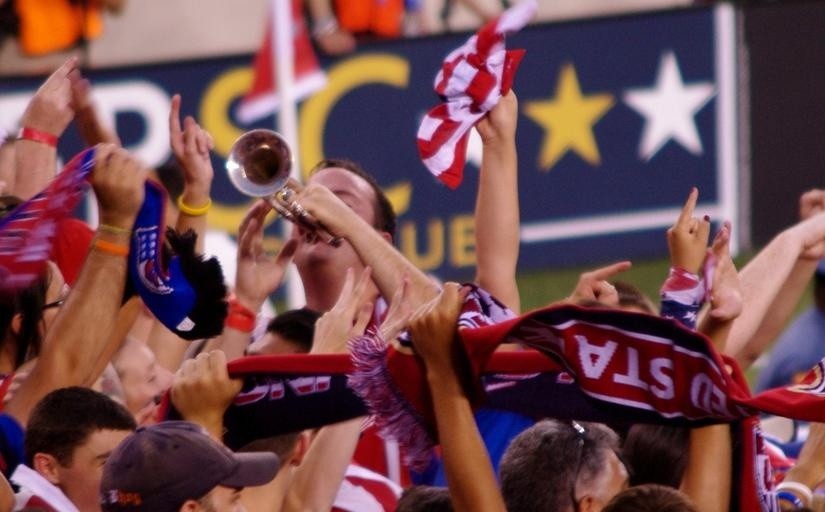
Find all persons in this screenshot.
[2,1,825,512]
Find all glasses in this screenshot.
[560,417,586,507]
[42,282,71,310]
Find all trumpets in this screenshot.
[225,130,342,250]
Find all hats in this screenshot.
[101,421,280,511]
